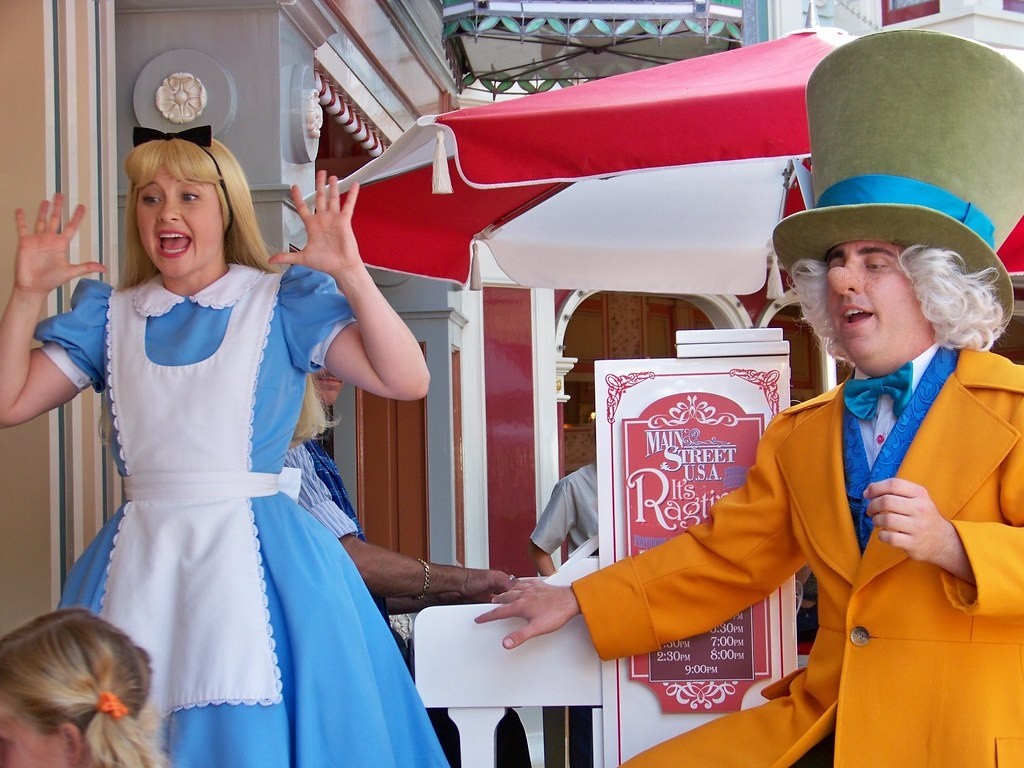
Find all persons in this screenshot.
[530,426,596,768]
[0,608,173,768]
[0,125,454,768]
[283,368,532,768]
[474,173,1024,768]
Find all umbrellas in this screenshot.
[302,27,1024,300]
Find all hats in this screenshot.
[773,30,1024,341]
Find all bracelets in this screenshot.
[414,559,430,600]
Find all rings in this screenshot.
[509,574,515,581]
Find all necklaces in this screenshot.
[797,595,818,619]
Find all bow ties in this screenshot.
[844,362,913,419]
